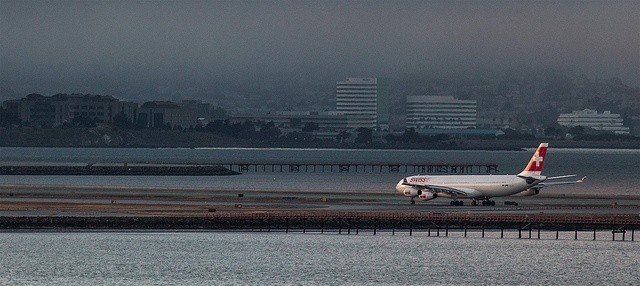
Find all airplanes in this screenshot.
[396,143,587,206]
